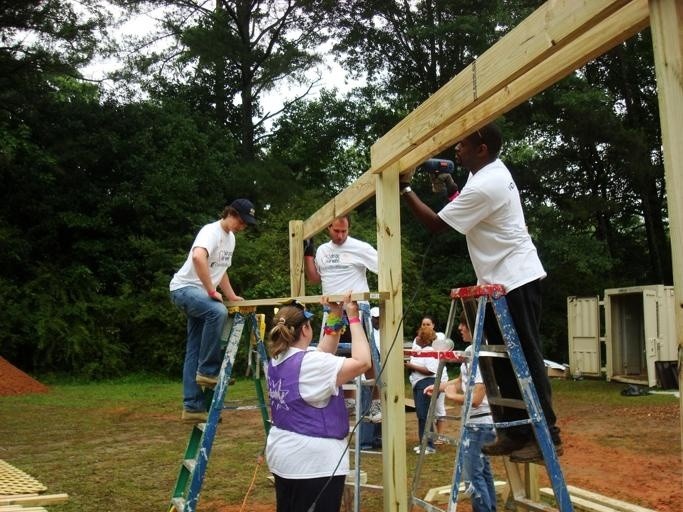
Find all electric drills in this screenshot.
[418,159,455,197]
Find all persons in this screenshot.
[423,310,498,511]
[359,305,381,451]
[399,121,565,461]
[404,326,443,455]
[411,314,450,446]
[168,197,260,424]
[264,288,372,512]
[302,213,381,424]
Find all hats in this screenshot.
[231,199,259,225]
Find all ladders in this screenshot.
[409,284,572,512]
[168,305,272,512]
[317,299,381,512]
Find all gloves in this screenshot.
[430,173,458,201]
[302,236,314,261]
[399,168,415,196]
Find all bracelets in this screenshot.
[400,185,412,196]
[322,311,349,337]
[304,255,314,260]
[348,317,362,324]
[448,190,460,202]
[208,287,217,297]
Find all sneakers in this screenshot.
[480,437,528,456]
[194,374,235,385]
[181,411,222,424]
[413,437,449,455]
[508,442,563,463]
